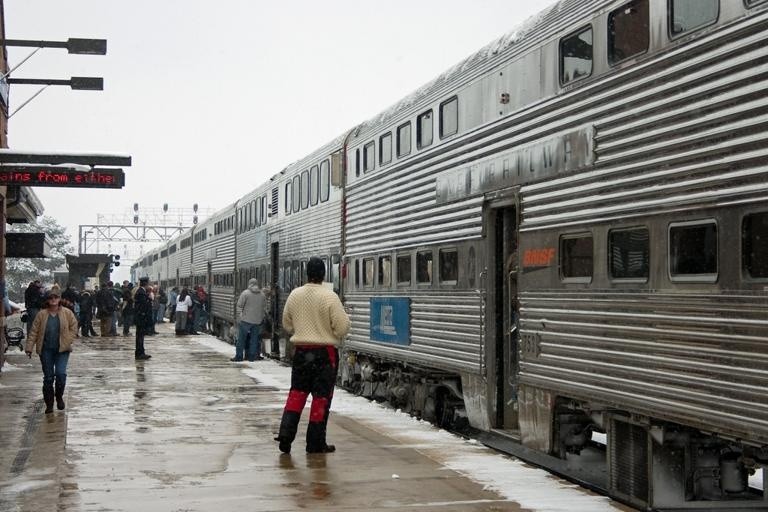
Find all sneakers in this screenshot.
[280,436,291,453]
[307,445,335,453]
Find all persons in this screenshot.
[231,277,271,362]
[24,278,207,360]
[273,258,353,454]
[25,283,78,414]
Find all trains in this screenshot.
[131,1,768,512]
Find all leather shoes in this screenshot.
[135,354,151,359]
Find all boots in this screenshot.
[55,383,65,409]
[43,380,54,413]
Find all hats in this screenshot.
[140,277,149,281]
[46,291,61,298]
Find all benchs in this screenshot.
[4,324,23,352]
